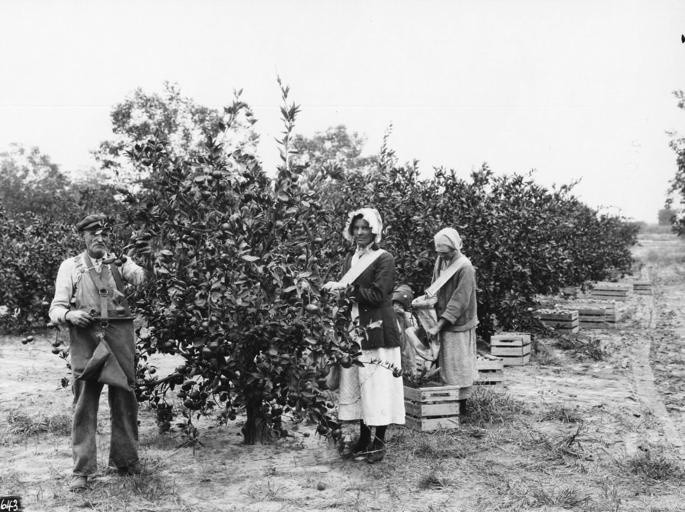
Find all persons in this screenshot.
[48,212,150,492]
[317,206,407,464]
[424,227,483,417]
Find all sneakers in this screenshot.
[349,438,387,463]
[69,475,87,491]
[115,464,153,474]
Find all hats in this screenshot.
[76,214,108,231]
[343,208,383,243]
